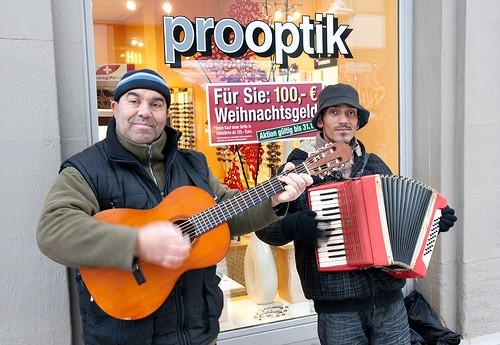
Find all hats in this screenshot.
[311,83,370,132]
[114,69,171,109]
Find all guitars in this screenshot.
[78,142,353,320]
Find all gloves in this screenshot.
[439,205,456,231]
[284,209,331,246]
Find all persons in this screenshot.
[34,68,315,345]
[254,82,457,345]
